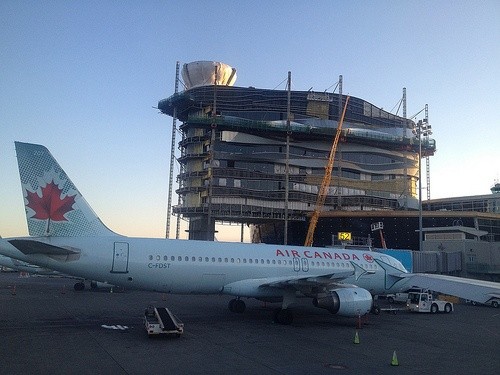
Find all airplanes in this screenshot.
[0,140,499,326]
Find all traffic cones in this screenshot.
[12,285,17,295]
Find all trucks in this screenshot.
[386,293,454,313]
